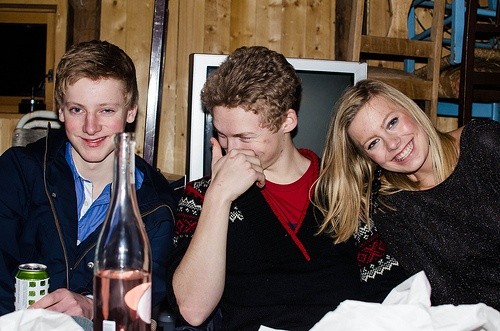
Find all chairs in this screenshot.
[12,110,60,148]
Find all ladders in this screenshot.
[458,0,500,131]
[347,0,446,131]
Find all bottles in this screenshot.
[93,130,152,331]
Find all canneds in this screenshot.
[14,263,49,312]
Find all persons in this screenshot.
[309,78,500,314]
[167,45,364,331]
[0,39,174,317]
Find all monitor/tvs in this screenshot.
[188,53,367,182]
